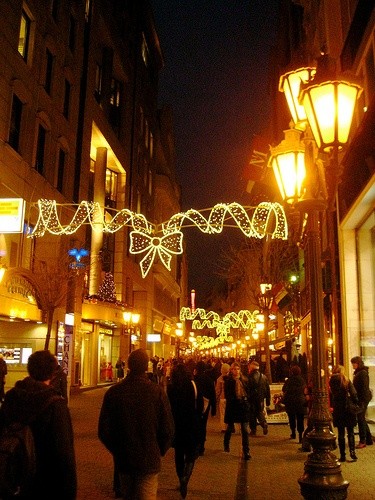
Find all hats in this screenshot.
[248,361,259,372]
[127,349,149,372]
[290,365,301,374]
[27,350,57,381]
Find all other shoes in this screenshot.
[180,477,188,499]
[350,450,357,459]
[365,441,373,445]
[262,423,268,435]
[339,456,345,461]
[249,431,256,436]
[356,441,365,449]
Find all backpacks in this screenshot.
[0,393,63,500]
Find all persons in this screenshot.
[0,350,77,500]
[100,353,373,500]
[97,348,177,500]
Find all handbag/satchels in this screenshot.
[203,398,209,414]
[346,396,364,414]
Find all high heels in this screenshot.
[290,434,295,439]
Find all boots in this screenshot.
[242,432,251,459]
[224,428,231,452]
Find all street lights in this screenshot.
[265,49,365,500]
[173,312,265,363]
[259,277,273,385]
[121,306,141,355]
